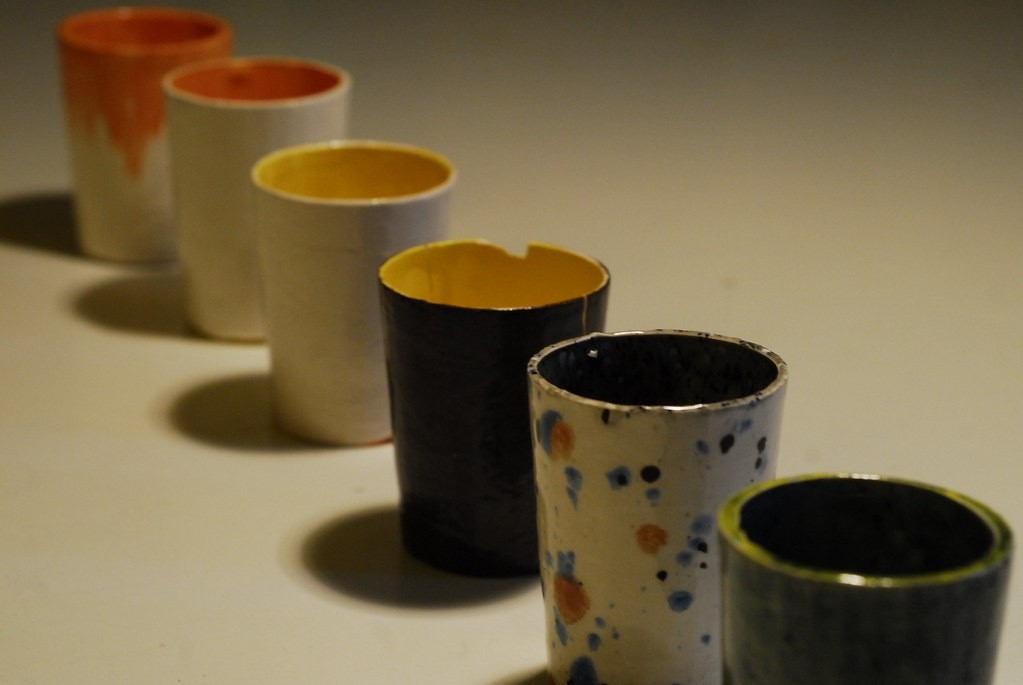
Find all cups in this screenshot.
[377,239,610,577]
[251,139,458,445]
[55,8,234,262]
[161,55,352,343]
[526,329,788,685]
[717,473,1013,685]
